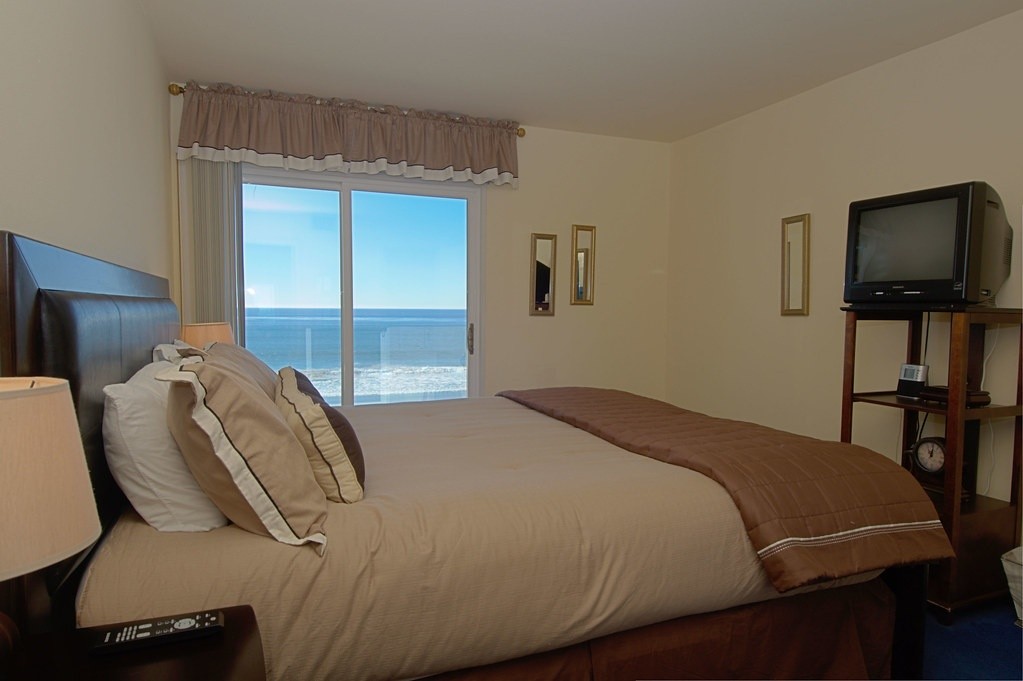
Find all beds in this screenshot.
[0,230,959,681]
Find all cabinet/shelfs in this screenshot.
[838,305,1023,628]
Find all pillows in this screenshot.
[153,339,200,363]
[102,361,229,532]
[203,341,277,402]
[153,346,329,558]
[274,365,366,504]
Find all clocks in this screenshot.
[908,435,971,504]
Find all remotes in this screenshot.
[101,610,227,648]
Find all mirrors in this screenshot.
[569,224,597,306]
[780,211,809,316]
[529,232,558,316]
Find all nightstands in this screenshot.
[0,605,266,681]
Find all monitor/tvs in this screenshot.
[843,181,1013,309]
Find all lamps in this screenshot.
[181,322,236,350]
[0,376,104,681]
